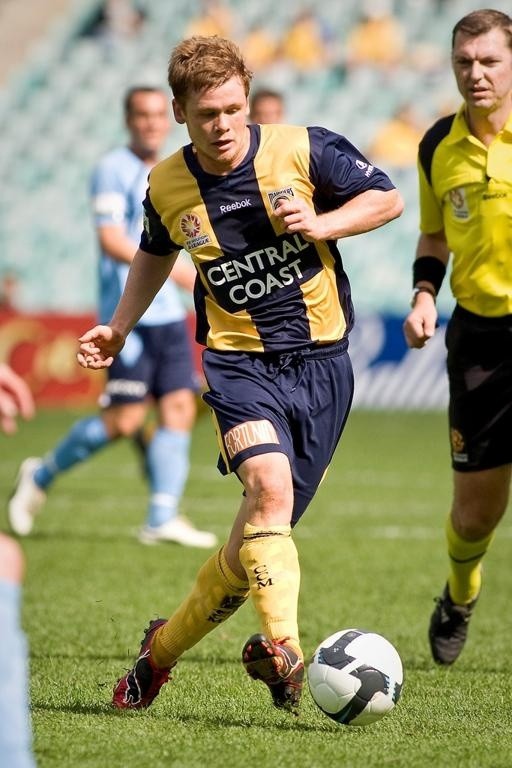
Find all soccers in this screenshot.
[307,629,403,725]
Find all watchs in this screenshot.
[409,286,436,310]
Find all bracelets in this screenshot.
[413,256,446,296]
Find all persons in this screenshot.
[255,1,328,72]
[338,1,408,70]
[8,87,219,549]
[402,9,512,665]
[368,100,429,166]
[0,361,37,768]
[184,2,278,75]
[250,91,285,125]
[75,33,404,712]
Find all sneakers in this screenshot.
[8,457,48,537]
[112,618,177,710]
[428,579,481,666]
[242,634,304,705]
[139,515,216,548]
